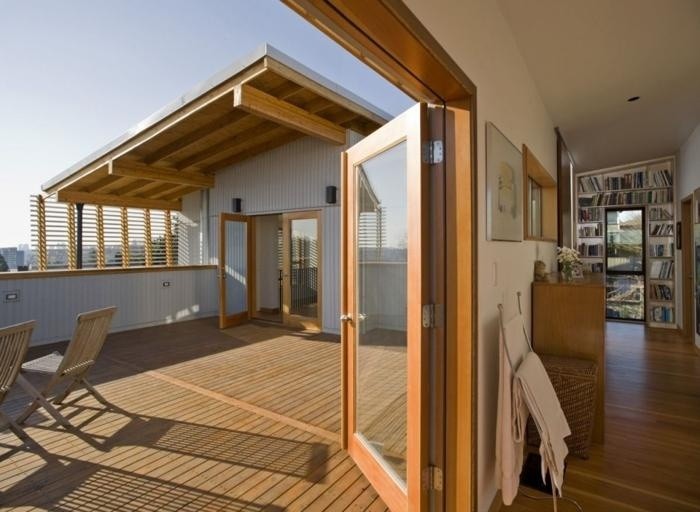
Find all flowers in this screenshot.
[556,248,581,265]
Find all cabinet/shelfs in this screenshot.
[575,155,677,325]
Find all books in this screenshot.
[601,169,676,205]
[578,173,602,274]
[644,204,675,323]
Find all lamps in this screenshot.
[326,185,336,203]
[232,198,241,213]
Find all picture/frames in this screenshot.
[485,121,522,242]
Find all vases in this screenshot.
[561,264,573,281]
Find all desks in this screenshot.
[532,273,605,445]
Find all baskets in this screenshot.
[525,352,600,462]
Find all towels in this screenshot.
[498,317,570,507]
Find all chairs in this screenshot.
[15,306,135,452]
[0,320,63,504]
